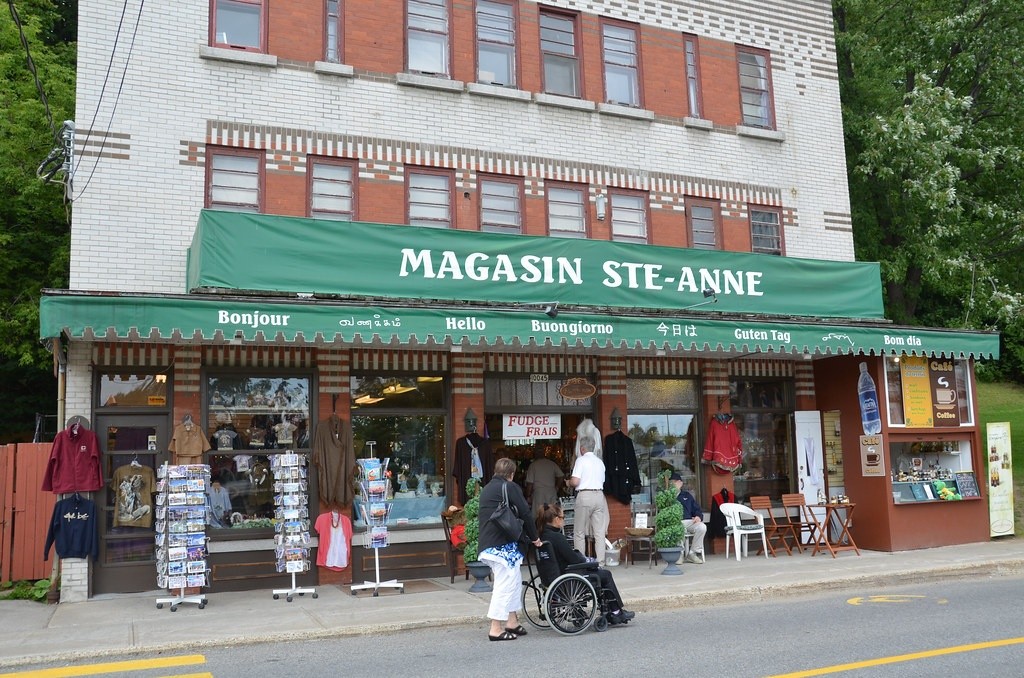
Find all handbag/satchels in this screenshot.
[491,482,522,541]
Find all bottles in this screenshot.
[817,489,824,505]
[857,361,881,435]
[909,461,913,475]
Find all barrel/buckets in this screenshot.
[605,550,620,567]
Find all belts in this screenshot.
[580,488,603,491]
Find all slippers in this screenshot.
[505,625,527,635]
[489,630,518,641]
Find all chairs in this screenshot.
[719,502,768,561]
[684,531,705,564]
[781,493,821,556]
[750,495,795,557]
[440,511,469,584]
[624,501,655,568]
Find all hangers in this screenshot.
[468,425,483,443]
[74,490,79,501]
[722,405,731,418]
[131,451,142,468]
[183,415,194,426]
[71,416,80,435]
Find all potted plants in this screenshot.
[464,477,492,592]
[657,470,682,574]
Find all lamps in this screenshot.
[465,407,477,432]
[515,301,559,318]
[671,288,717,314]
[595,193,606,220]
[609,407,622,430]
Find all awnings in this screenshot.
[38,288,999,366]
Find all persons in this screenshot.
[669,473,707,565]
[564,436,609,567]
[494,446,574,538]
[475,457,543,642]
[219,455,280,527]
[651,440,667,457]
[535,503,636,625]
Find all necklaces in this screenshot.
[331,512,340,528]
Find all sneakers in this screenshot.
[687,553,703,564]
[608,608,635,624]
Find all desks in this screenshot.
[804,502,861,558]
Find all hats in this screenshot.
[668,473,683,481]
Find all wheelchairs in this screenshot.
[520,538,632,636]
